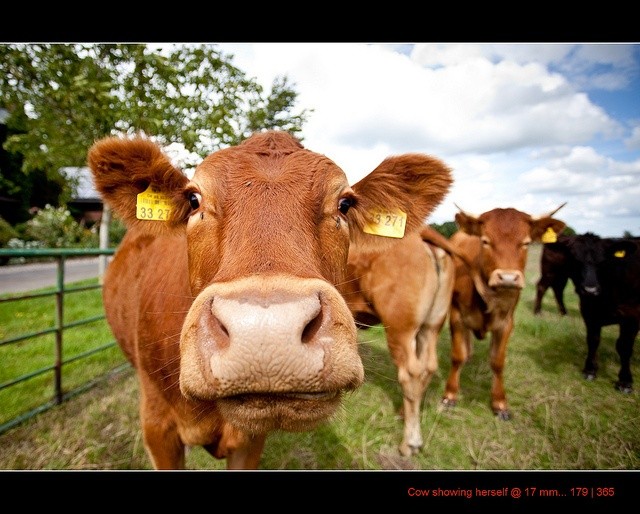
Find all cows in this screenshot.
[546,232,640,394]
[533,234,575,315]
[347,225,474,459]
[86,130,456,471]
[441,202,569,421]
